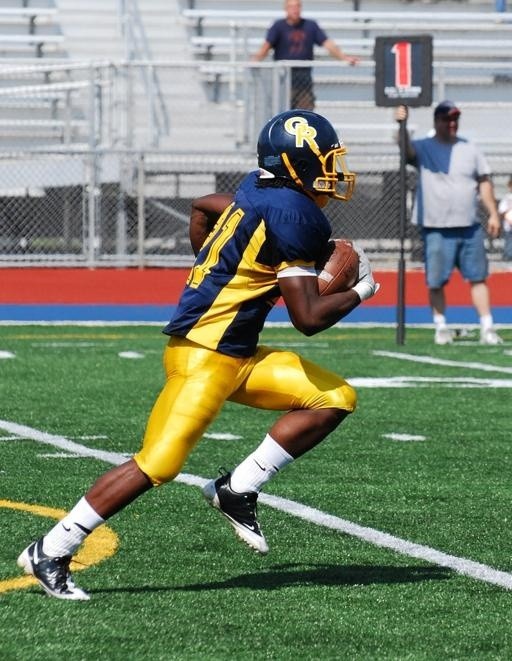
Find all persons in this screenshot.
[406,166,425,263]
[17,109,373,600]
[395,100,504,346]
[249,0,360,113]
[498,181,512,262]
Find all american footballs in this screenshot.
[314,240,359,296]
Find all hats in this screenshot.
[434,98,461,120]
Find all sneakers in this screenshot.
[16,536,92,603]
[434,324,454,346]
[200,464,271,557]
[479,325,504,347]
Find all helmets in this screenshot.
[255,108,356,203]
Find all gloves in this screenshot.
[351,243,381,296]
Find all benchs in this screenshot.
[184,0,512,175]
[0,1,85,151]
